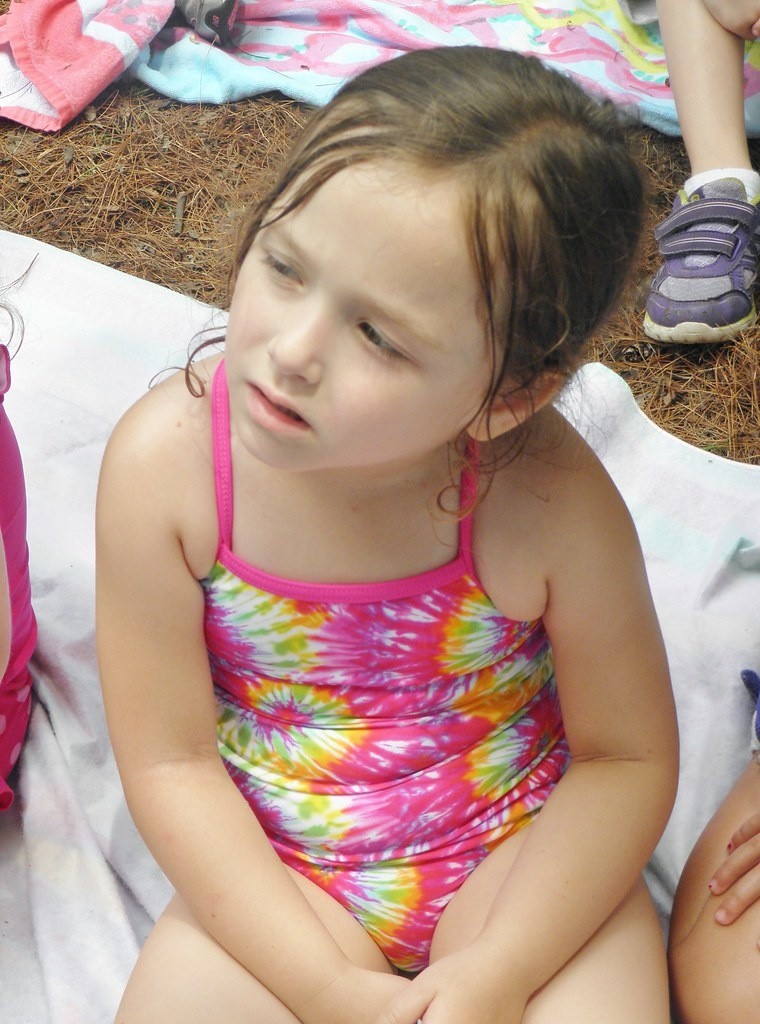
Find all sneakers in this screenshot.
[644,177,760,345]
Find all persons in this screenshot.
[97,44,679,1024]
[0,344,37,810]
[667,670,760,1024]
[642,0,760,345]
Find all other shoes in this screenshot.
[175,0,239,45]
